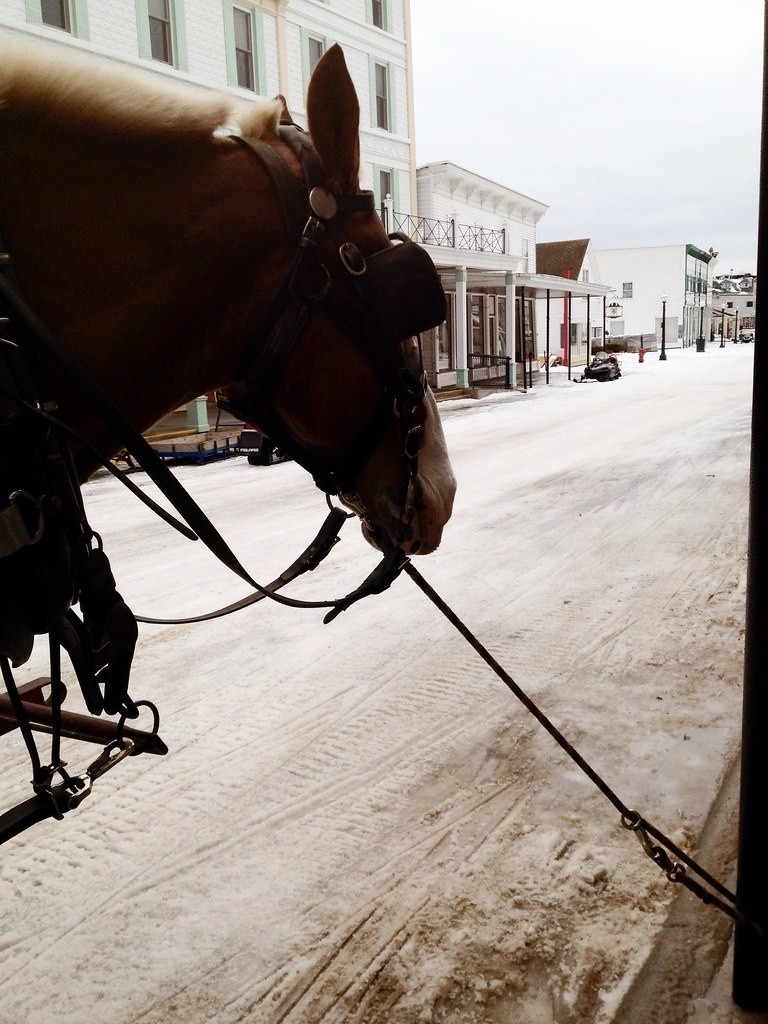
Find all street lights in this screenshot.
[734,306,739,343]
[659,293,668,360]
[719,303,726,348]
[699,300,706,352]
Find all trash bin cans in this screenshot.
[696,339,706,352]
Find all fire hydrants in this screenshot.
[638,349,646,362]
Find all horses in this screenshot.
[0,38,458,560]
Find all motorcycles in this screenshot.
[583,351,622,382]
